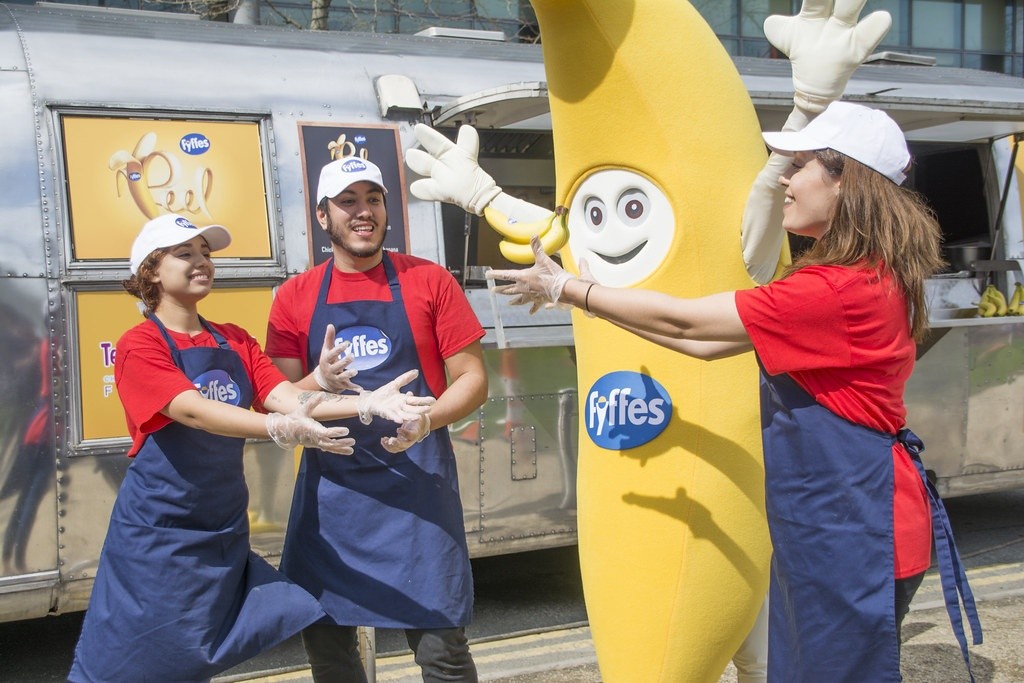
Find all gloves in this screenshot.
[406,123,503,217]
[763,0,892,112]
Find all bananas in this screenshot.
[970,281,1023,316]
[109,133,180,221]
[483,1,793,683]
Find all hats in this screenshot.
[762,100,911,188]
[130,213,231,275]
[316,157,388,206]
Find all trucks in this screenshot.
[1,0,1024,622]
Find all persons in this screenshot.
[484,102,988,682]
[262,156,487,682]
[60,211,437,683]
[2,292,66,576]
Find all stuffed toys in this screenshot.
[402,0,897,680]
